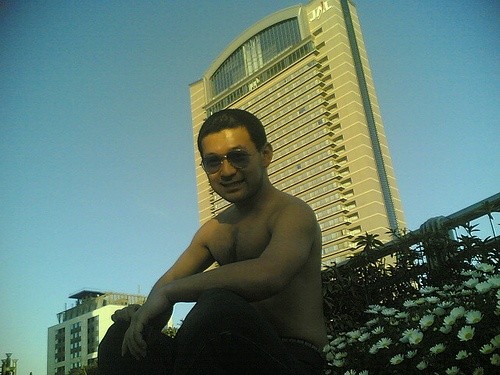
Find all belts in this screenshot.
[280,337,323,357]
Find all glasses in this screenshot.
[199,147,268,177]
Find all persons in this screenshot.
[96,108,331,375]
